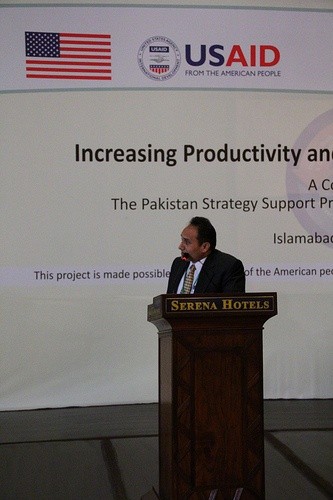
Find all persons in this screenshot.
[165,215,247,295]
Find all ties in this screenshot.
[181,265,196,294]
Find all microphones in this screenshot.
[184,253,220,293]
